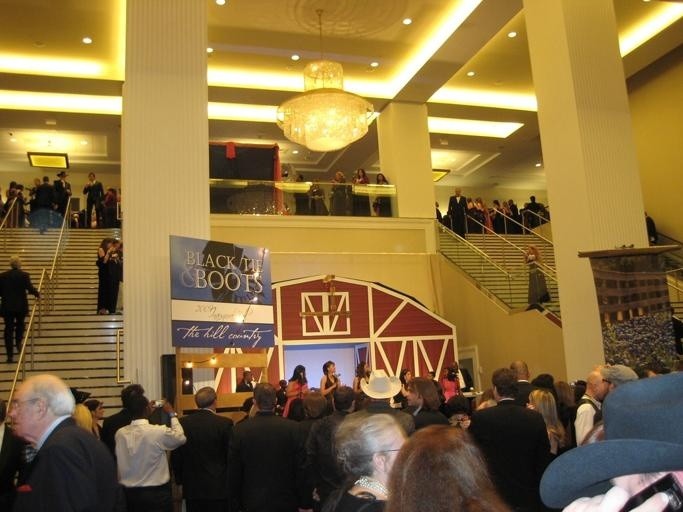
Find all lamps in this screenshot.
[275,9,375,154]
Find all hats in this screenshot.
[540,369,683,510]
[601,365,639,385]
[57,172,68,177]
[361,369,402,399]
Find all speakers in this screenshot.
[161,354,177,405]
[181,368,193,394]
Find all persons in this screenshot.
[434,188,546,237]
[526,245,551,306]
[107,239,123,313]
[96,238,114,314]
[0,256,40,363]
[644,211,657,243]
[291,169,390,215]
[0,171,119,228]
[1,360,683,512]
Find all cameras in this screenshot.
[152,400,165,408]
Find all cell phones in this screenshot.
[619,473,683,512]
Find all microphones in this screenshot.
[300,372,303,379]
[332,373,337,378]
